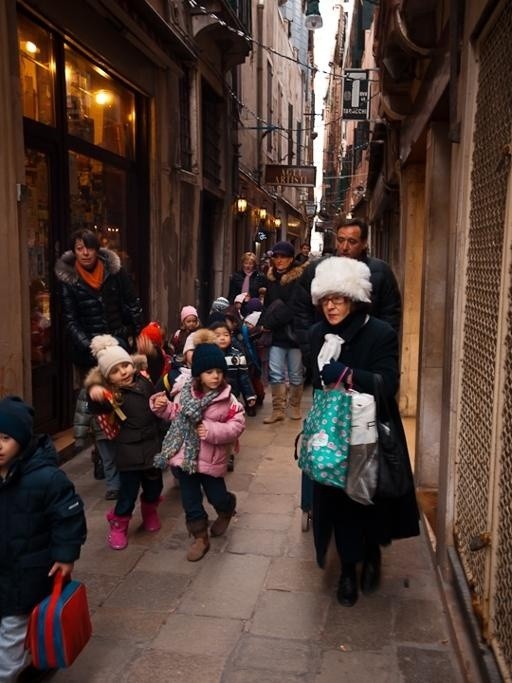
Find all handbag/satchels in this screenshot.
[29,580,92,669]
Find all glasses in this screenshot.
[319,295,353,306]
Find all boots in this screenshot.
[211,491,236,537]
[107,510,131,549]
[263,381,288,423]
[185,515,210,562]
[289,382,304,420]
[140,492,161,532]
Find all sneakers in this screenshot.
[227,455,233,472]
[256,392,264,405]
[92,450,105,479]
[244,404,256,417]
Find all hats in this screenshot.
[183,333,196,356]
[90,334,134,380]
[204,296,240,335]
[310,256,373,307]
[234,292,262,327]
[271,241,294,257]
[192,329,227,378]
[139,321,162,346]
[0,396,36,450]
[181,306,198,323]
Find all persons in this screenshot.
[297,256,422,606]
[207,243,316,423]
[54,229,145,480]
[295,216,403,532]
[1,394,88,683]
[73,305,257,562]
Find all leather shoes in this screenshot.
[360,553,380,594]
[106,490,119,500]
[337,571,357,607]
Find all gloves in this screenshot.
[321,358,351,387]
